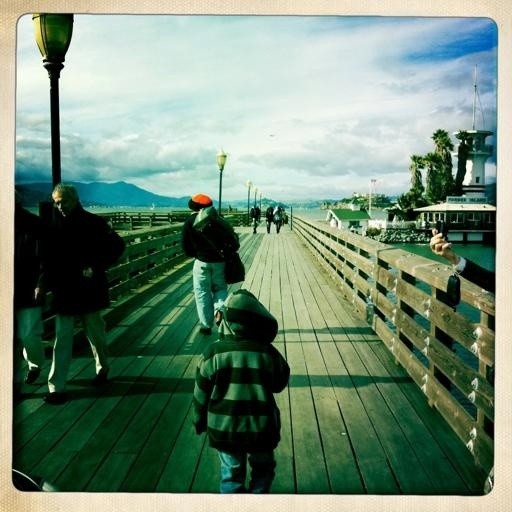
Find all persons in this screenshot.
[182,193,232,335]
[249,203,261,234]
[272,205,284,234]
[264,206,274,233]
[426,228,495,293]
[39,182,126,404]
[189,288,289,493]
[15,182,47,386]
[280,206,286,227]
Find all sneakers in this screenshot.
[21,363,110,405]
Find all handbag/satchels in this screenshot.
[220,248,248,286]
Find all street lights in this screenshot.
[216,150,227,215]
[32,14,74,190]
[246,180,263,225]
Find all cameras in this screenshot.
[435,220,448,240]
